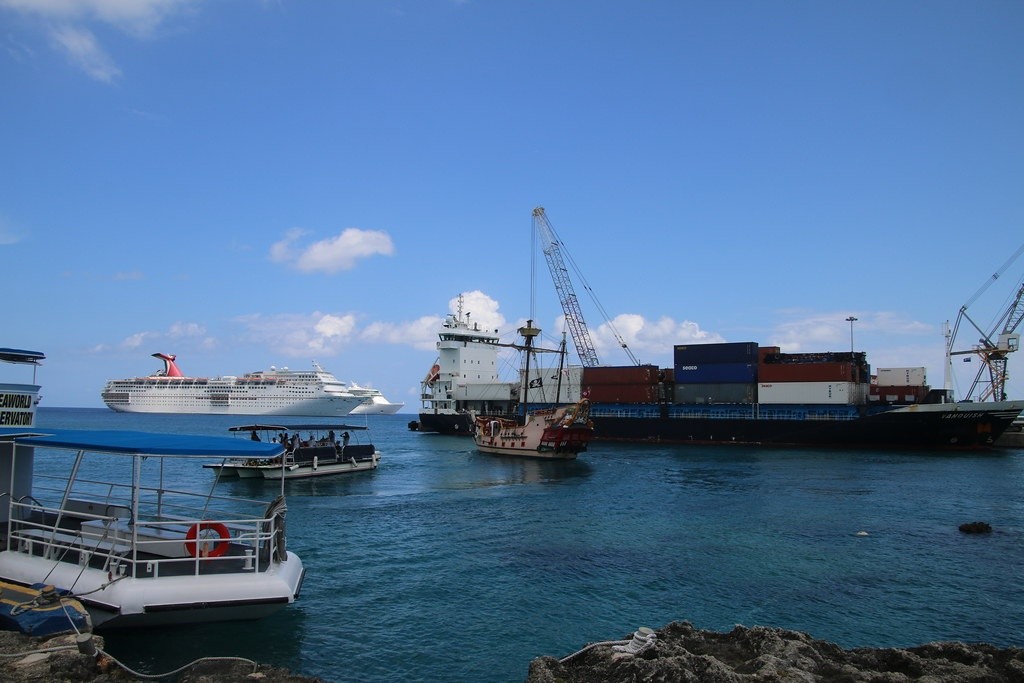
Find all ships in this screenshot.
[98,350,372,418]
[417,314,1024,448]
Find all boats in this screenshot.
[0,346,311,641]
[207,420,384,486]
[339,380,405,413]
[467,401,597,462]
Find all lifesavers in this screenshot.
[484,427,490,435]
[186,519,230,562]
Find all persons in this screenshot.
[250,430,351,455]
